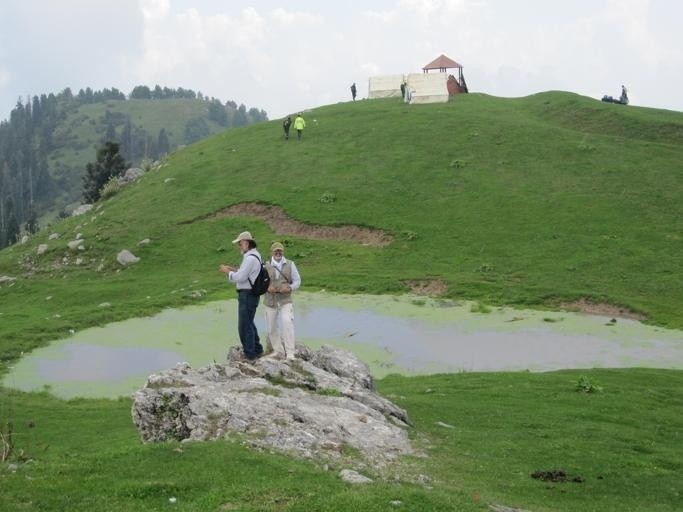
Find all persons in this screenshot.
[281,115,292,141]
[348,81,358,103]
[263,241,302,363]
[217,230,266,365]
[400,82,405,98]
[292,113,307,142]
[600,84,629,106]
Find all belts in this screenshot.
[238,289,249,292]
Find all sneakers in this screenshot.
[257,352,265,357]
[239,356,257,364]
[286,354,296,362]
[265,352,285,360]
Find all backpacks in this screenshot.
[248,254,270,296]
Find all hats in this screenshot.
[232,231,253,244]
[271,242,284,251]
